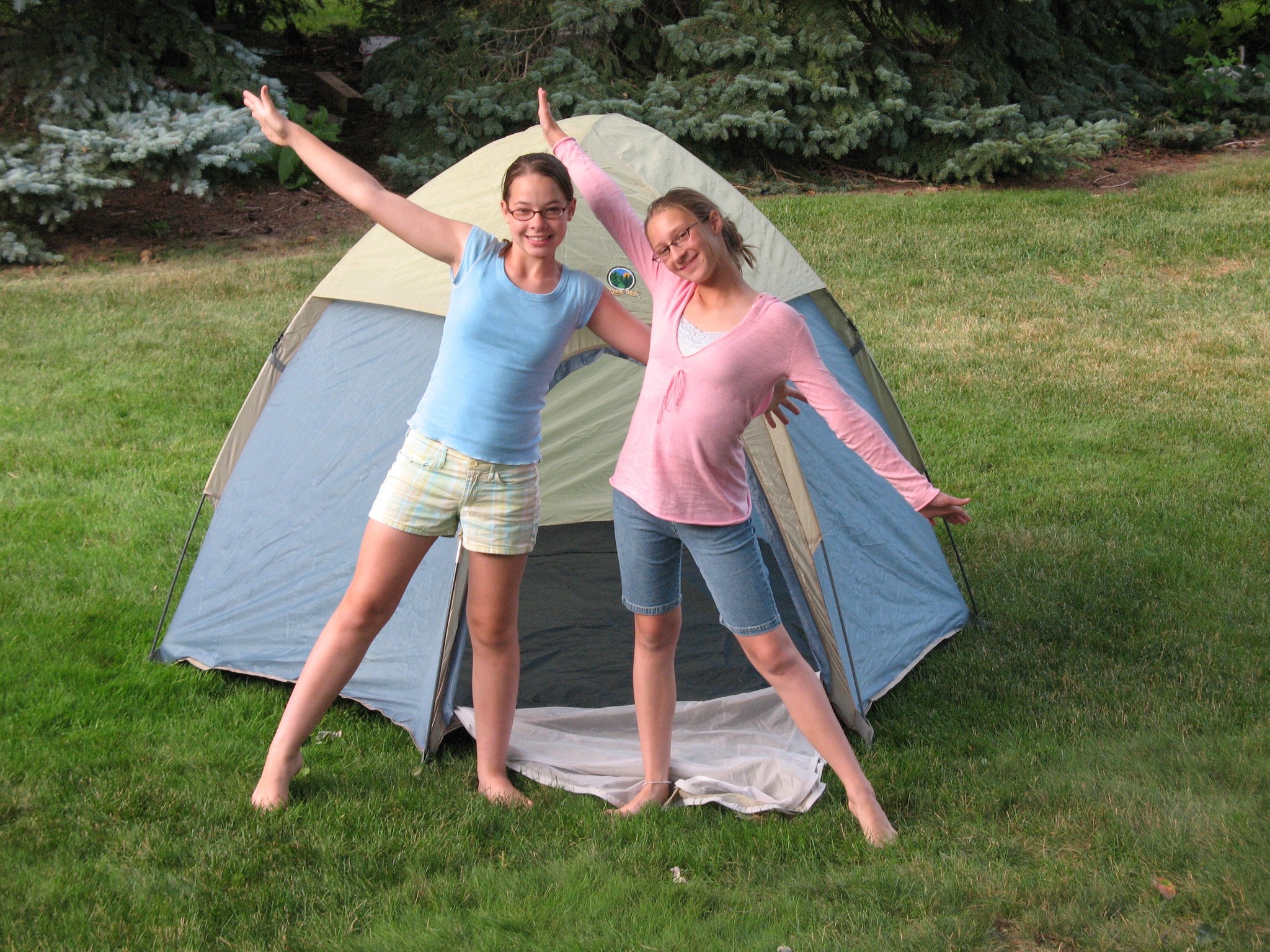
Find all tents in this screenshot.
[148,114,978,818]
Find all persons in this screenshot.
[240,85,813,814]
[538,87,972,850]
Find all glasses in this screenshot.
[506,200,570,221]
[652,216,708,263]
